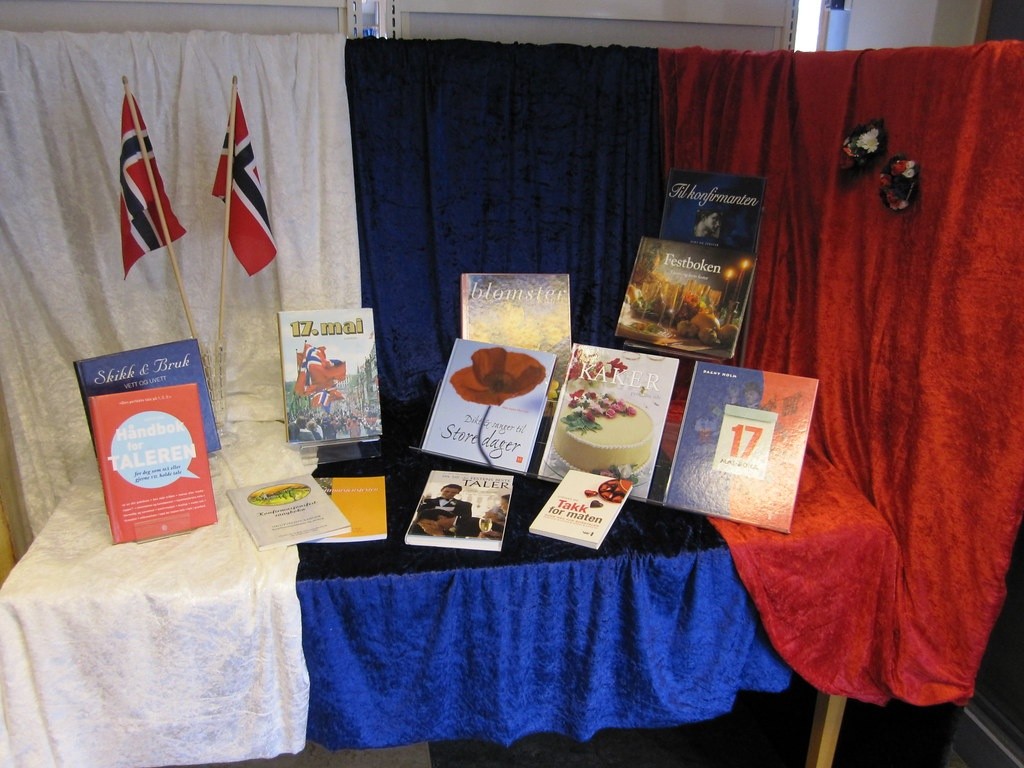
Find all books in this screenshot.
[226,473,352,551]
[87,383,219,542]
[422,338,557,475]
[405,471,514,552]
[460,273,572,416]
[662,168,766,251]
[304,476,387,542]
[538,343,679,503]
[614,234,756,360]
[278,308,382,445]
[529,470,632,550]
[73,338,222,454]
[662,360,820,532]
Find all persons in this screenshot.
[409,484,511,540]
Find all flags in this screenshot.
[212,93,276,278]
[120,92,186,281]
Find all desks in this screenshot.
[0,416,895,768]
